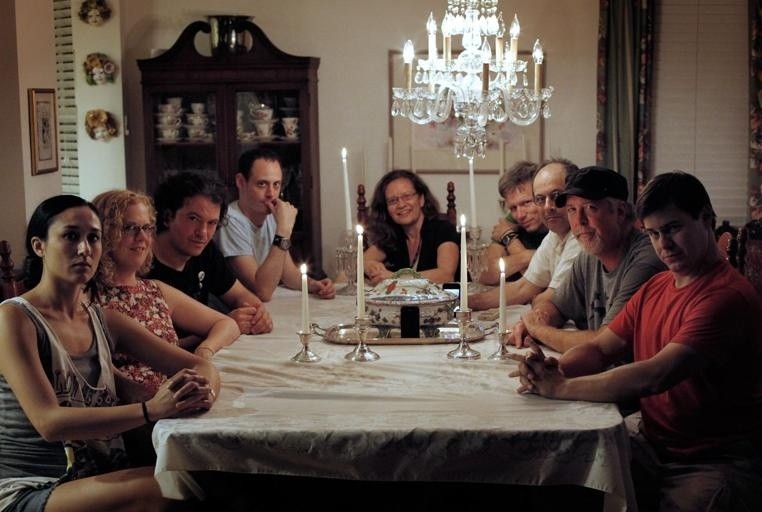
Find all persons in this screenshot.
[137,167,275,335]
[503,171,762,511]
[478,158,548,288]
[362,168,474,286]
[503,165,669,356]
[90,190,242,466]
[1,191,225,511]
[457,160,580,315]
[79,1,118,145]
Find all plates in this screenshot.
[276,135,300,141]
[254,136,278,142]
[248,118,279,124]
[279,106,297,115]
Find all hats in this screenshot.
[554,166,629,208]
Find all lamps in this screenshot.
[390,0,554,161]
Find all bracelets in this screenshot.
[140,398,151,423]
[497,228,519,246]
[196,346,215,355]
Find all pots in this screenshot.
[350,269,459,337]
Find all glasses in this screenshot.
[533,190,564,207]
[124,222,155,235]
[385,192,416,205]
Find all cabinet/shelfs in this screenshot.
[136,13,322,280]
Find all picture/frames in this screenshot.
[386,47,546,174]
[26,87,60,176]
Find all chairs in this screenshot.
[358,181,456,228]
[736,217,762,292]
[715,220,737,267]
[0,240,34,297]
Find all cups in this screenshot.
[285,98,297,107]
[155,97,209,146]
[280,117,300,137]
[251,104,273,119]
[255,123,272,136]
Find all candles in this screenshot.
[354,223,368,318]
[458,212,469,311]
[341,146,354,232]
[300,260,312,332]
[498,257,507,334]
[468,157,479,228]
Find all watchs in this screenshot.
[271,234,292,251]
[213,147,339,300]
